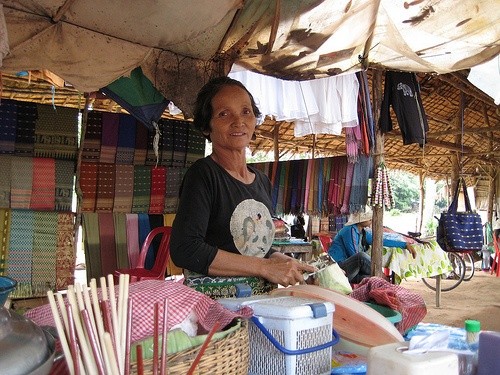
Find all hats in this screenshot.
[345,204,372,226]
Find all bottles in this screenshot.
[465,320,481,344]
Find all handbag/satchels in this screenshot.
[436,178,484,254]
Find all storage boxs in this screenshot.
[218,295,336,375]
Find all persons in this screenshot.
[328,203,416,284]
[169,76,315,287]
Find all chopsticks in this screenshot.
[46,273,222,374]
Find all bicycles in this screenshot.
[407,216,474,292]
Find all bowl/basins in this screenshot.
[0,275,17,308]
[193,317,241,346]
[361,301,403,326]
[129,328,197,361]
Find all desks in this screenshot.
[367,239,453,307]
[331,322,481,375]
[272,241,314,285]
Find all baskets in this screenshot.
[130,318,250,375]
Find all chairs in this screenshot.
[112,226,173,285]
